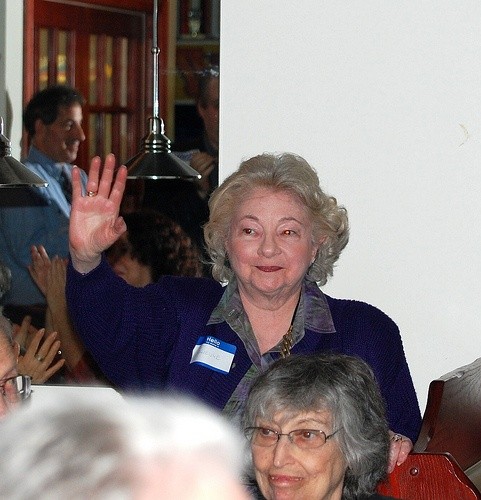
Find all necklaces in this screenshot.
[281,291,302,359]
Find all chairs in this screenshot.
[375,357,481,500]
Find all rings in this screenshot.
[35,354,44,363]
[392,433,402,443]
[86,190,98,197]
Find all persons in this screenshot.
[67,151,422,474]
[1,69,248,500]
[242,353,392,500]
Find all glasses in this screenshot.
[241,425,344,451]
[1,375,31,409]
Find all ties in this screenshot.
[61,168,74,206]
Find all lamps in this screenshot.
[112,14,202,182]
